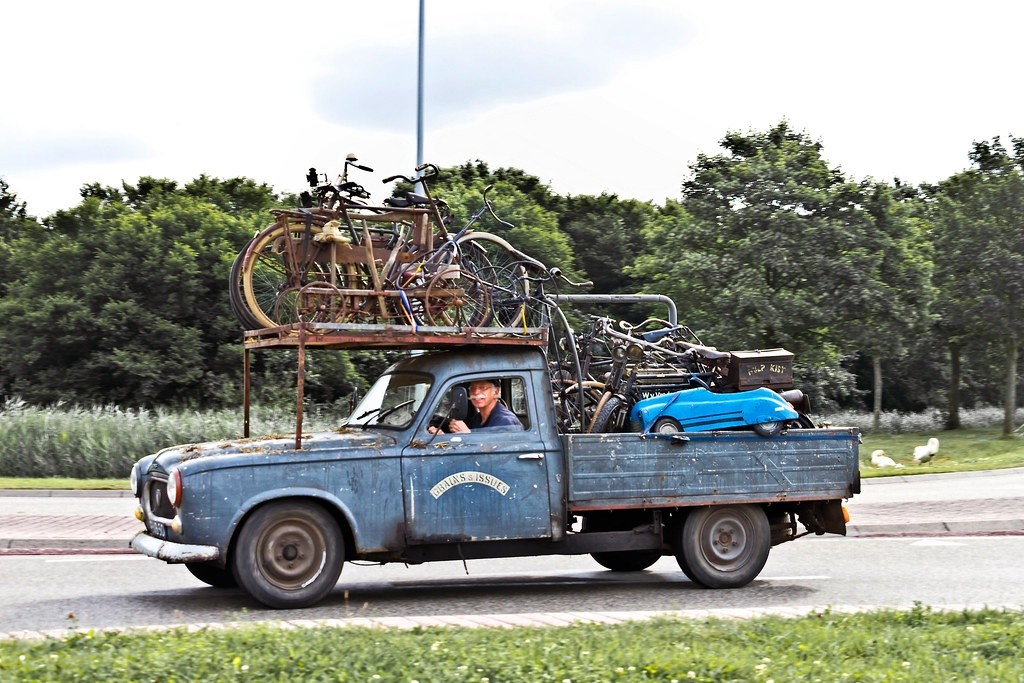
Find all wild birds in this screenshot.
[871,438,944,468]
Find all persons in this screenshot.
[428,377,526,438]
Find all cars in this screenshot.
[631,386,799,437]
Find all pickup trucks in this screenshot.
[127,348,861,611]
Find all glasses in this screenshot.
[468,384,496,391]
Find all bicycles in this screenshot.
[229,153,731,434]
